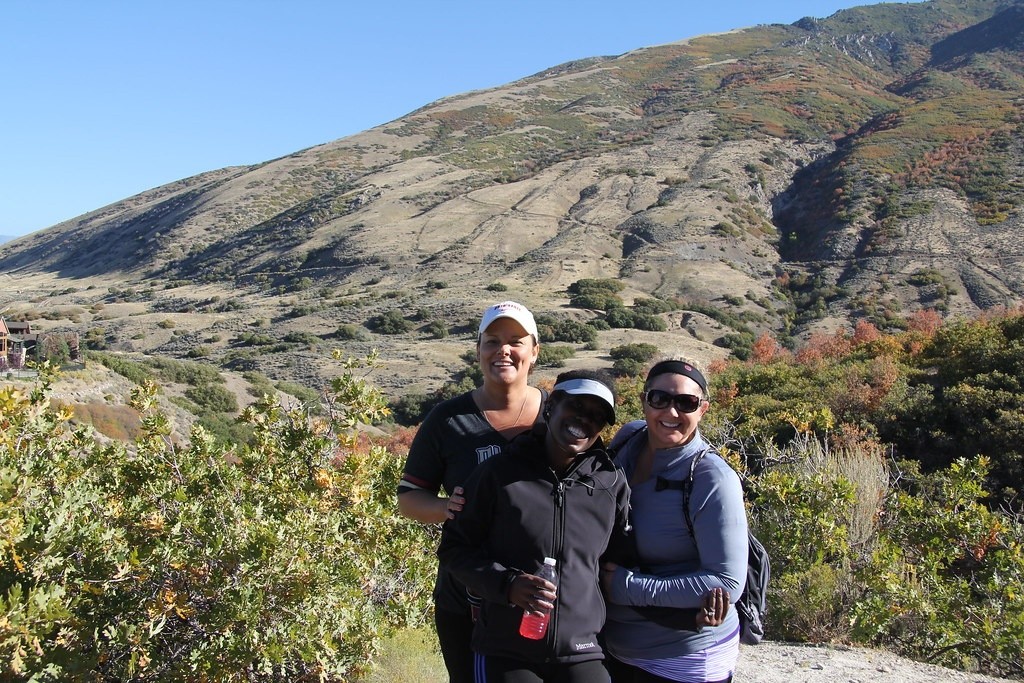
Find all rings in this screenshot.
[708,611,715,616]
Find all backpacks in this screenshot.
[609,424,771,645]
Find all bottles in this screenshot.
[518,557,559,640]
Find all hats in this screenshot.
[477,300,538,345]
[552,377,617,427]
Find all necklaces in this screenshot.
[484,388,529,430]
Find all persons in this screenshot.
[438,368,730,683]
[395,299,550,662]
[602,356,750,683]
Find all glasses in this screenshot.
[643,389,704,413]
